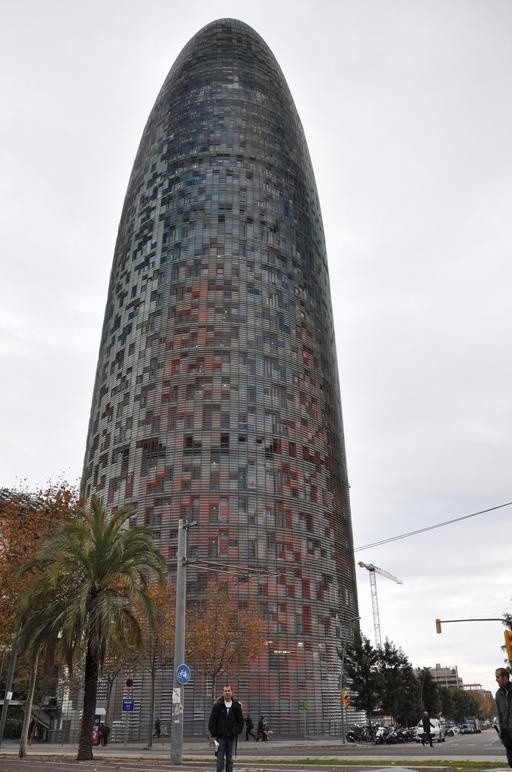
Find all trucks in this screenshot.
[463,716,481,734]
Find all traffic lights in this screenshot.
[341,690,348,703]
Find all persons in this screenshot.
[208,684,244,772]
[96,722,111,746]
[421,710,436,747]
[153,718,163,738]
[494,667,512,766]
[245,716,273,742]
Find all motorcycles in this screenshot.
[346,723,416,744]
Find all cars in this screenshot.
[415,719,445,743]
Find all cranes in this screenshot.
[358,561,403,651]
